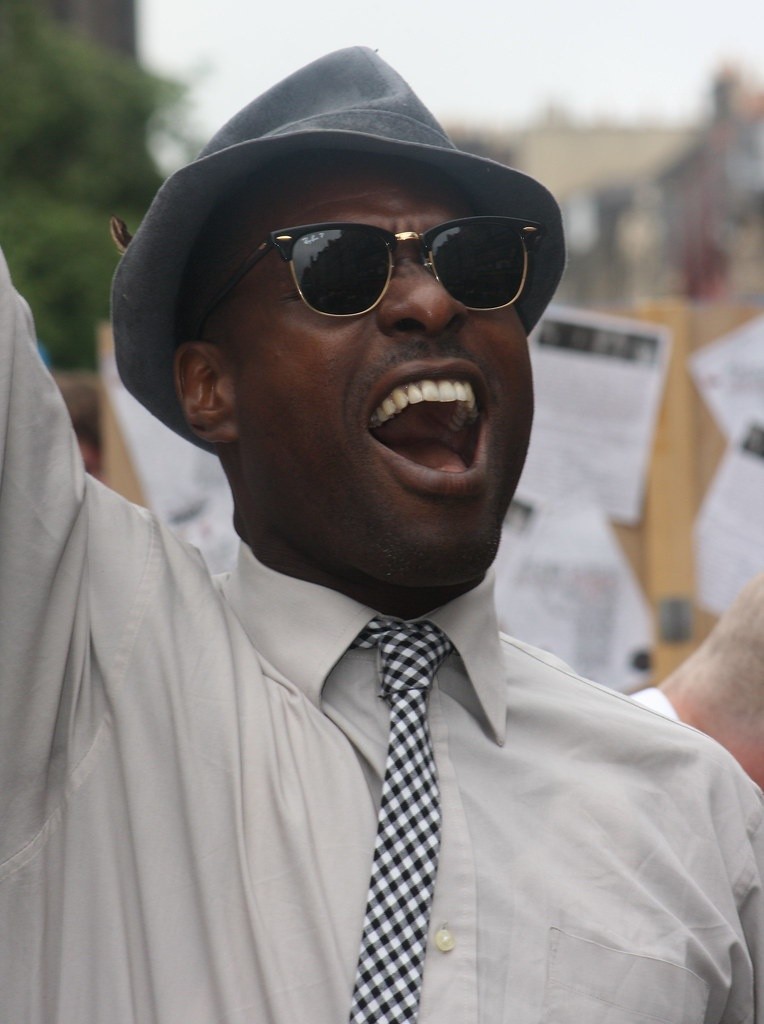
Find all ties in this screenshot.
[346,616,455,1024]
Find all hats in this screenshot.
[108,45,566,458]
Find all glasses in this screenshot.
[210,215,548,321]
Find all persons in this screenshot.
[0,45,764,1024]
